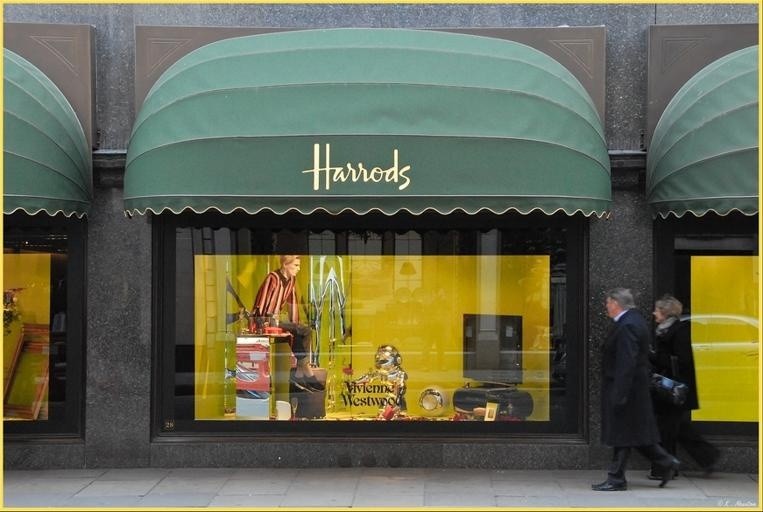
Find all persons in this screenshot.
[592,287,720,491]
[248,255,325,394]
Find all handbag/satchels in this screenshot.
[648,372,688,404]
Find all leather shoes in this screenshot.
[702,459,723,476]
[591,474,628,491]
[648,458,680,487]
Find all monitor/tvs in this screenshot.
[463,312,522,392]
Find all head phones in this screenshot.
[378,346,402,366]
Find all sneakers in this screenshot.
[290,374,325,393]
